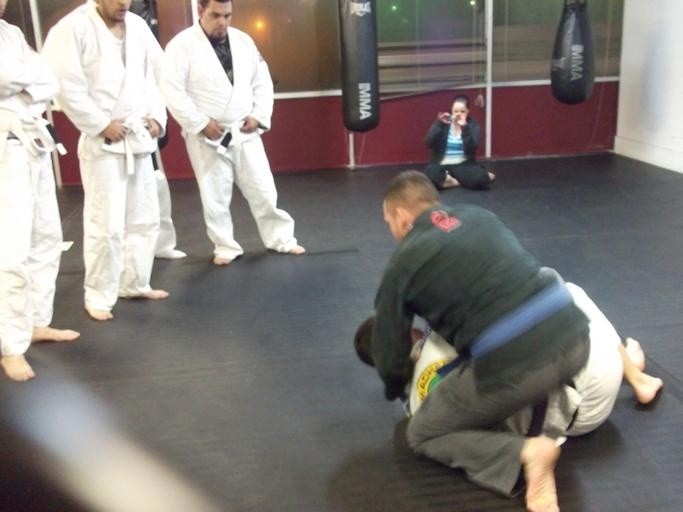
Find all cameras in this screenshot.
[448,115,456,122]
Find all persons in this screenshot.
[1,1,81,382]
[354,282,665,442]
[44,0,188,322]
[371,170,591,512]
[424,95,496,190]
[162,1,305,266]
